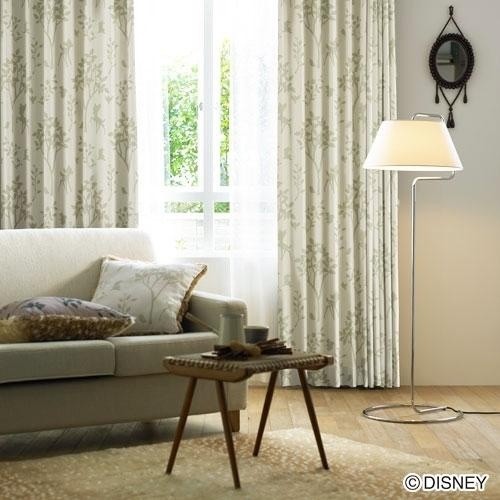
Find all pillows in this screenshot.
[92,256,208,335]
[1,296,136,343]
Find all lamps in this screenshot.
[362,113,465,425]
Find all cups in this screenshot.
[245,326,269,343]
[218,312,244,348]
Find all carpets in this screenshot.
[1,426,500,499]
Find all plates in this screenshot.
[201,352,217,357]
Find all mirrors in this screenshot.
[431,9,474,126]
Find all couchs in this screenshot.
[1,228,247,434]
